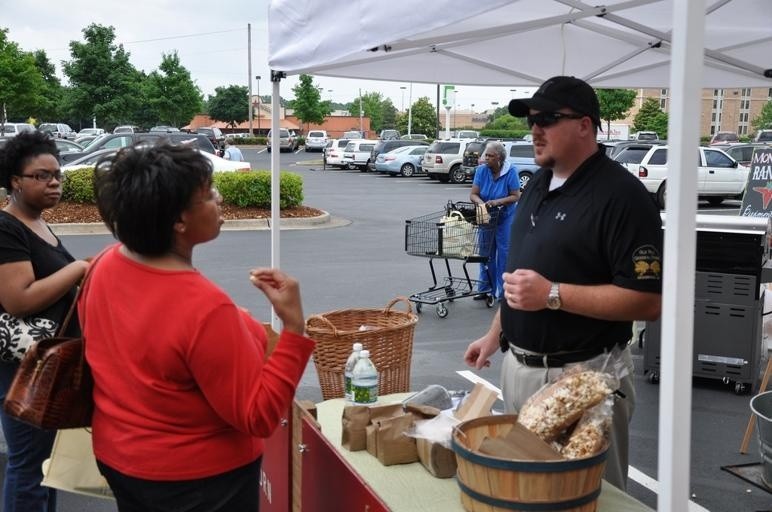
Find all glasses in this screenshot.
[527,112,583,126]
[16,173,67,184]
[177,186,217,210]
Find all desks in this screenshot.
[258,370,654,512]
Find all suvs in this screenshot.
[0,123,250,166]
[600,129,772,208]
[266,128,298,151]
[421,140,547,191]
[323,139,351,169]
[305,130,329,151]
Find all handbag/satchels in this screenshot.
[1,336,93,430]
[436,211,480,257]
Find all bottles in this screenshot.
[344,343,362,405]
[351,350,378,408]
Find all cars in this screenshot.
[403,134,430,139]
[58,146,251,173]
[376,145,429,177]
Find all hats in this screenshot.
[508,76,602,131]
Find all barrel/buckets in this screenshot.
[750,391,772,491]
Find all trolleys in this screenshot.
[405,200,504,317]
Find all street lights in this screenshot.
[318,86,333,102]
[254,75,262,133]
[399,86,526,116]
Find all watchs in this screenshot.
[546,281,561,311]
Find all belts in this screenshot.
[507,341,617,367]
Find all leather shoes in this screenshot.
[473,294,488,300]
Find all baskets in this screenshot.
[303,296,419,401]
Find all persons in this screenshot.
[222,137,244,162]
[0,132,91,512]
[469,142,520,301]
[464,76,662,491]
[75,140,316,511]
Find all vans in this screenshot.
[345,139,431,173]
[457,130,478,141]
[378,130,400,139]
